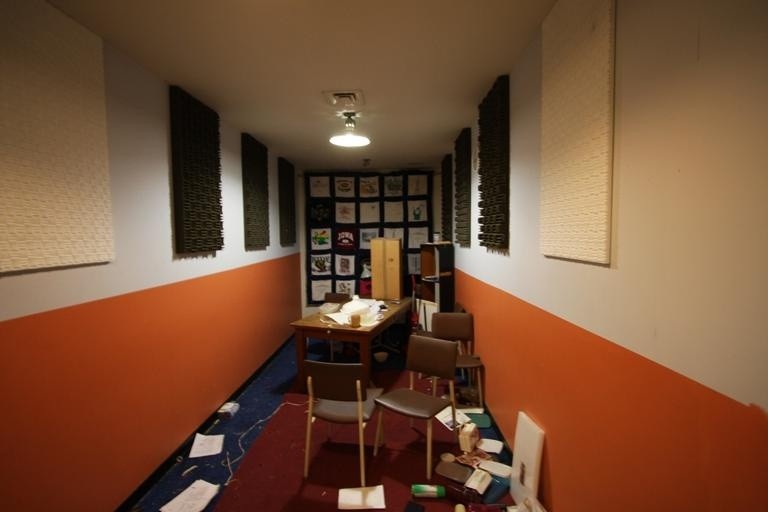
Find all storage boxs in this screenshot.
[457,422,480,453]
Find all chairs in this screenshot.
[303,293,351,361]
[298,357,383,487]
[432,312,485,406]
[374,334,461,481]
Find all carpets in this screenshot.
[206,342,516,510]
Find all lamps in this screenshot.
[329,111,371,150]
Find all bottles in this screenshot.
[410,483,444,498]
[432,231,440,243]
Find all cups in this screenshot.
[347,312,362,327]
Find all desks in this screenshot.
[290,296,414,388]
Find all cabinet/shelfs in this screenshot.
[370,235,404,301]
[418,237,453,313]
